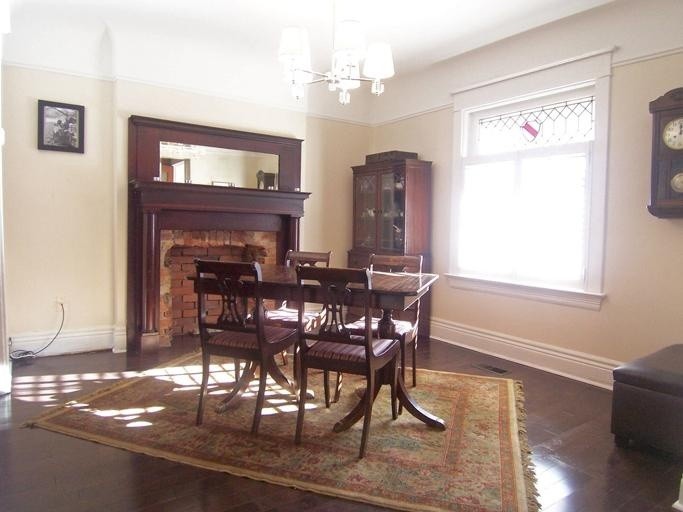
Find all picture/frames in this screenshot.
[36,99,85,155]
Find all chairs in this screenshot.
[196,259,302,432]
[294,263,399,456]
[275,249,332,364]
[333,253,424,414]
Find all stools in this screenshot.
[610,343,682,467]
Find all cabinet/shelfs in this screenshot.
[349,150,433,341]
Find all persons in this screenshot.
[48,116,78,147]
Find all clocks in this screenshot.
[648,86,683,219]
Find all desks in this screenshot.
[185,263,445,431]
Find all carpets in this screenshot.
[24,348,541,512]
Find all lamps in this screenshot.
[276,19,396,106]
